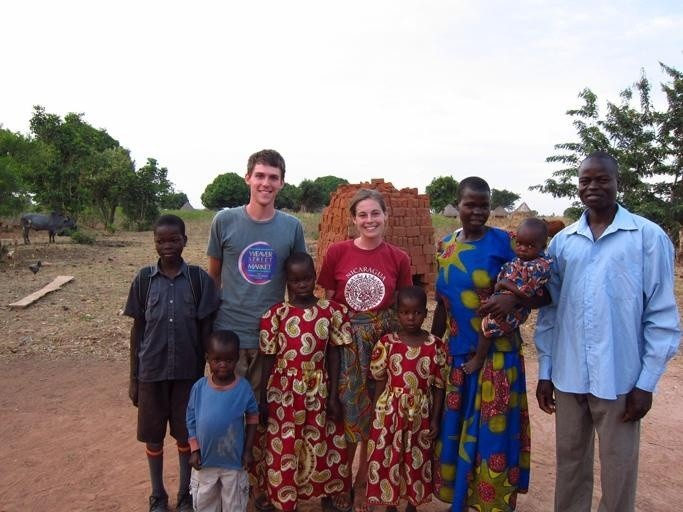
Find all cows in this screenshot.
[20,212,77,245]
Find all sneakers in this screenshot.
[149,491,168,512]
[176,492,194,512]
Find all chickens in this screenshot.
[28,260,42,276]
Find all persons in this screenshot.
[203,147,307,412]
[181,328,264,512]
[316,188,413,512]
[121,213,221,512]
[370,284,448,512]
[428,174,555,512]
[530,151,683,512]
[460,216,555,377]
[243,249,357,512]
